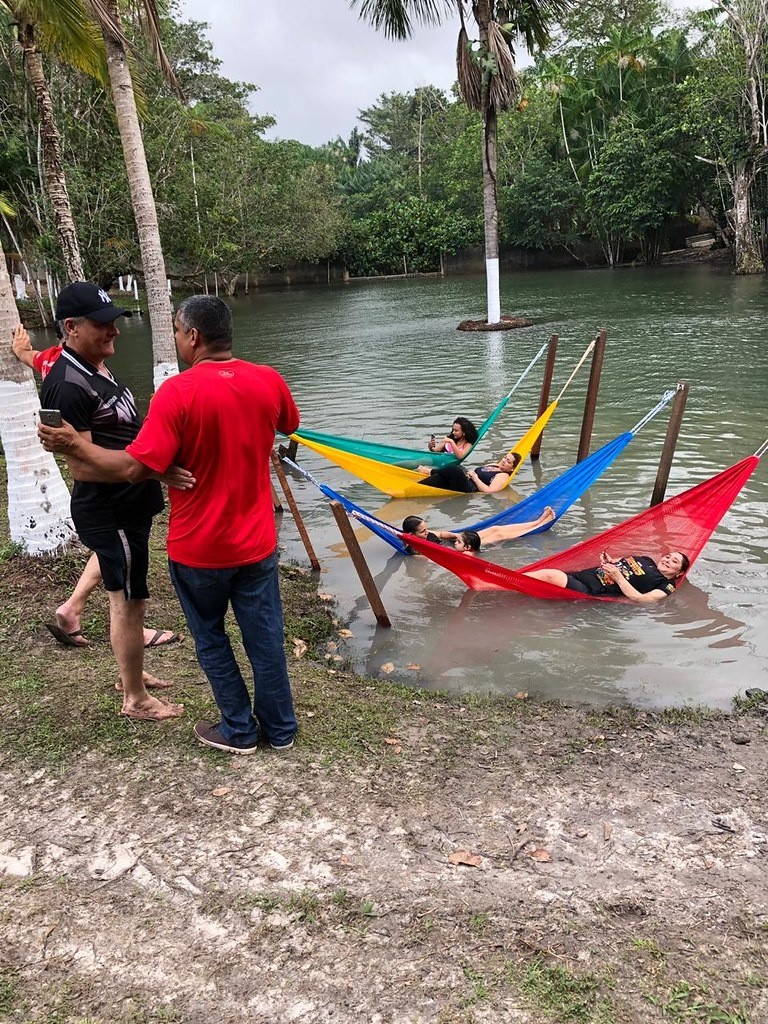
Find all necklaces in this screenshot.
[198,356,233,362]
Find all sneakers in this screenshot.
[193,722,257,755]
[261,728,294,749]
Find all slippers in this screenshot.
[144,628,177,648]
[45,621,91,647]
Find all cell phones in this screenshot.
[39,409,62,429]
[430,433,436,448]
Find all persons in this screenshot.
[37,296,300,754]
[454,532,482,556]
[13,324,176,647]
[391,417,479,470]
[402,506,555,556]
[41,283,196,721]
[388,452,522,497]
[468,551,690,603]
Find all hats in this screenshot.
[55,281,132,321]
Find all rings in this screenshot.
[42,440,46,444]
